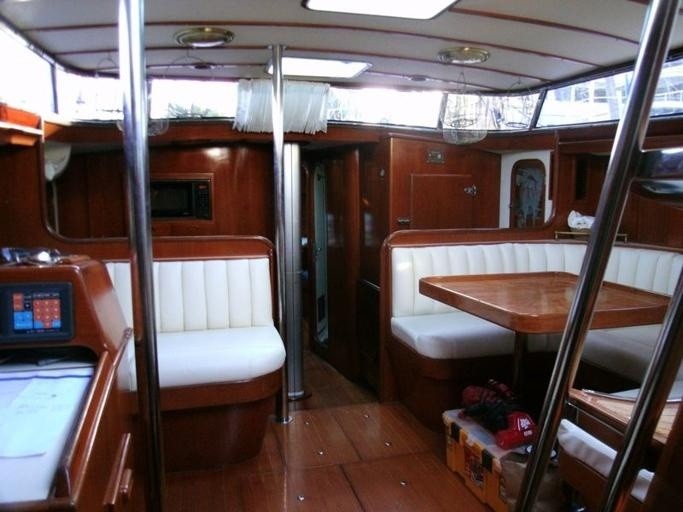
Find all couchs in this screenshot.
[384,239,682,512]
[98,257,288,471]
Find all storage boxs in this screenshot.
[442,409,497,505]
[481,444,559,512]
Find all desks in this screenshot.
[418,271,671,409]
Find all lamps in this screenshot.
[436,47,490,65]
[173,27,234,49]
